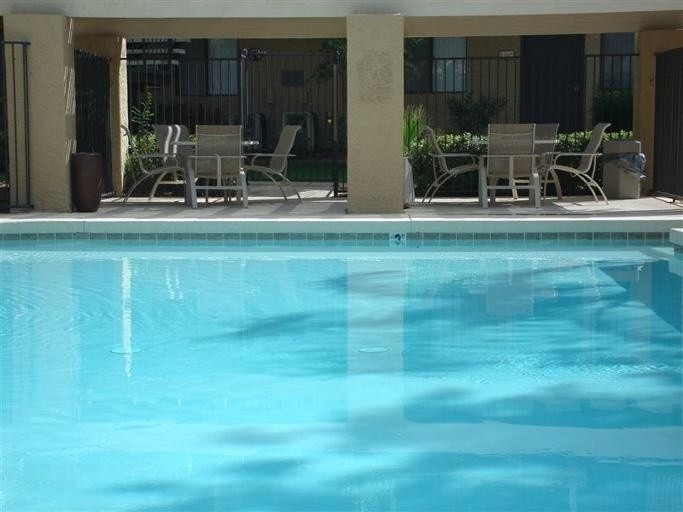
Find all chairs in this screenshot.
[119,123,302,209]
[419,122,611,208]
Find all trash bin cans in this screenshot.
[602,140,642,199]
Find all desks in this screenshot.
[316,146,346,197]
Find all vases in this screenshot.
[69,152,104,213]
